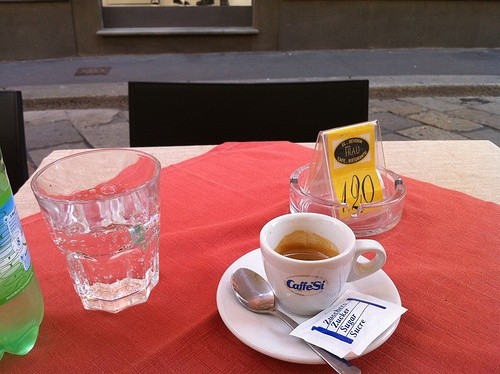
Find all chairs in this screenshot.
[127,79,370,148]
[0,90,29,196]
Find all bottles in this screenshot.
[0,143,43,361]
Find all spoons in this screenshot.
[230,268,362,374]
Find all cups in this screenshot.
[30,148,161,314]
[259,212,386,316]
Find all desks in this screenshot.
[0,139,500,374]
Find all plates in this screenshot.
[215,249,402,364]
